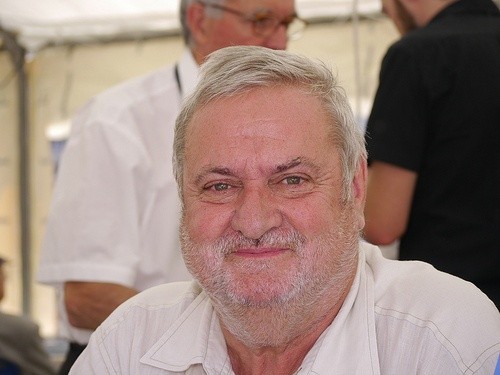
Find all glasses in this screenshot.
[203,3,308,39]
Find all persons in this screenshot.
[360,0,500,311]
[66,45,500,375]
[0,257,55,375]
[36,1,310,375]
